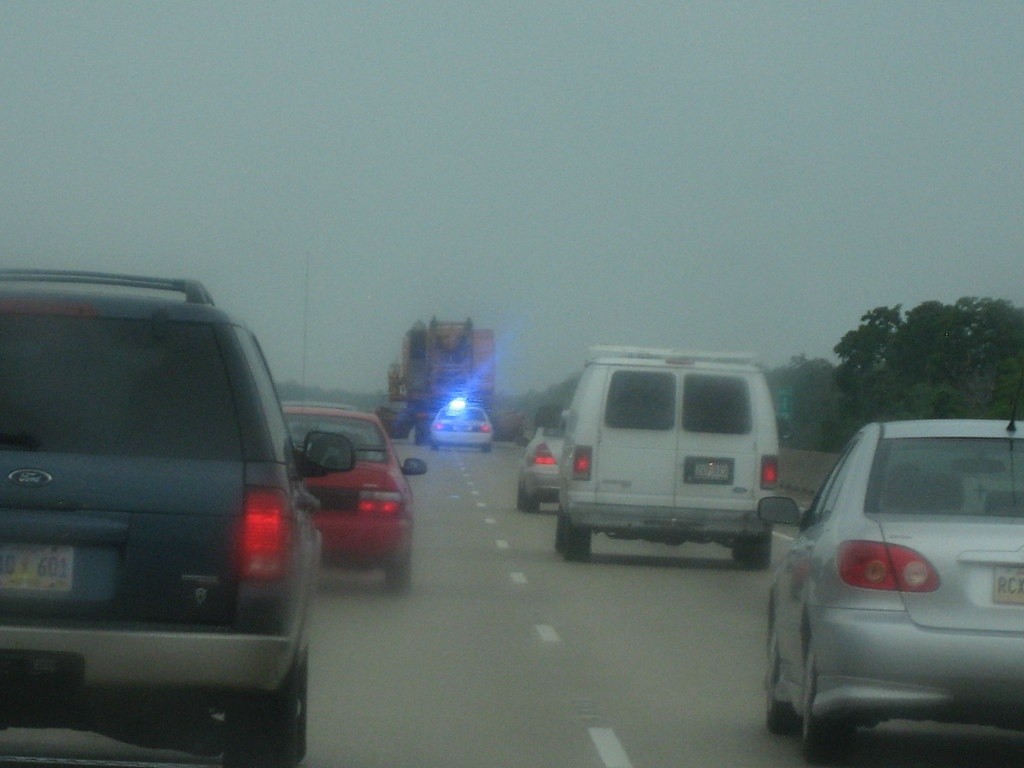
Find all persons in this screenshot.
[883,462,933,511]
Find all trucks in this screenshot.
[376,309,522,445]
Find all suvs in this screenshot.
[0,270,355,768]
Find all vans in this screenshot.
[556,346,780,563]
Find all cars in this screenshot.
[515,428,565,509]
[427,400,494,451]
[758,418,1024,768]
[283,407,427,593]
